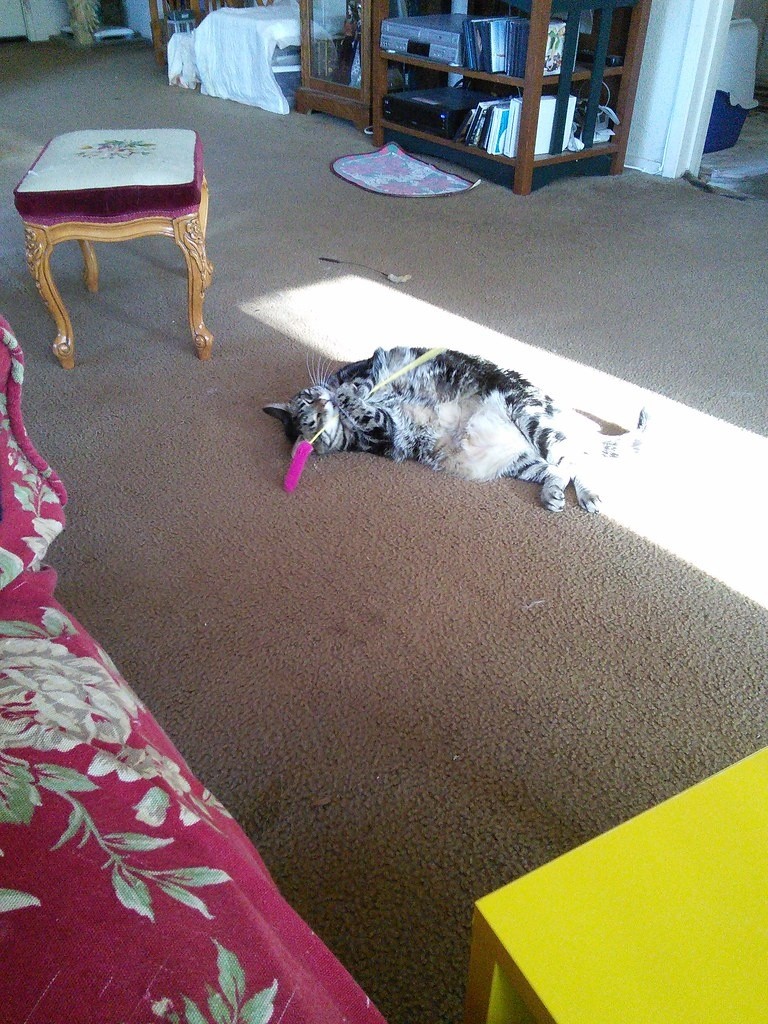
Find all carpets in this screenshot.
[330,141,482,198]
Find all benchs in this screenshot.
[1,316,387,1024]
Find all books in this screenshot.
[462,16,581,78]
[453,99,511,155]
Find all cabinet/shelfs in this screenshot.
[372,0,651,197]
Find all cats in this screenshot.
[263,347,649,513]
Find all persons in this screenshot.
[546,26,566,59]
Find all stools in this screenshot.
[13,129,214,360]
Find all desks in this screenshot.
[469,744,767,1023]
